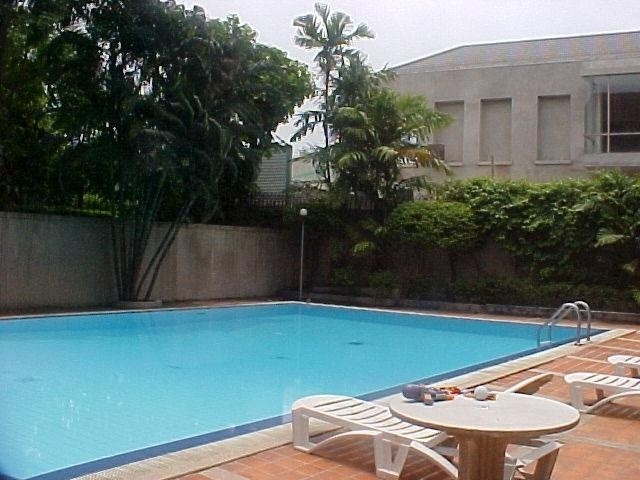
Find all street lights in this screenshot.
[296,208,308,302]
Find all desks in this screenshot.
[385,386,583,480]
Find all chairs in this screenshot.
[287,371,556,480]
[562,355,640,415]
